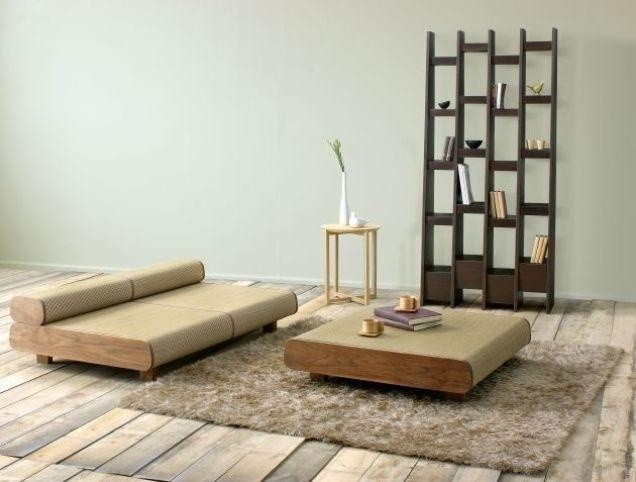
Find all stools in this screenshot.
[10,259,298,381]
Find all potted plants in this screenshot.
[326,136,349,225]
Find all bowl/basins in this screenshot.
[437,100,451,110]
[464,138,483,149]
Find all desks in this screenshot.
[323,224,379,306]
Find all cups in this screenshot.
[399,294,414,311]
[361,317,379,335]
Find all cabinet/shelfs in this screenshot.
[420,26,557,315]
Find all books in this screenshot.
[371,315,443,331]
[374,305,443,326]
[490,190,508,219]
[491,82,508,109]
[457,164,474,206]
[530,234,549,264]
[441,136,456,162]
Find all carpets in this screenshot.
[118,302,622,475]
